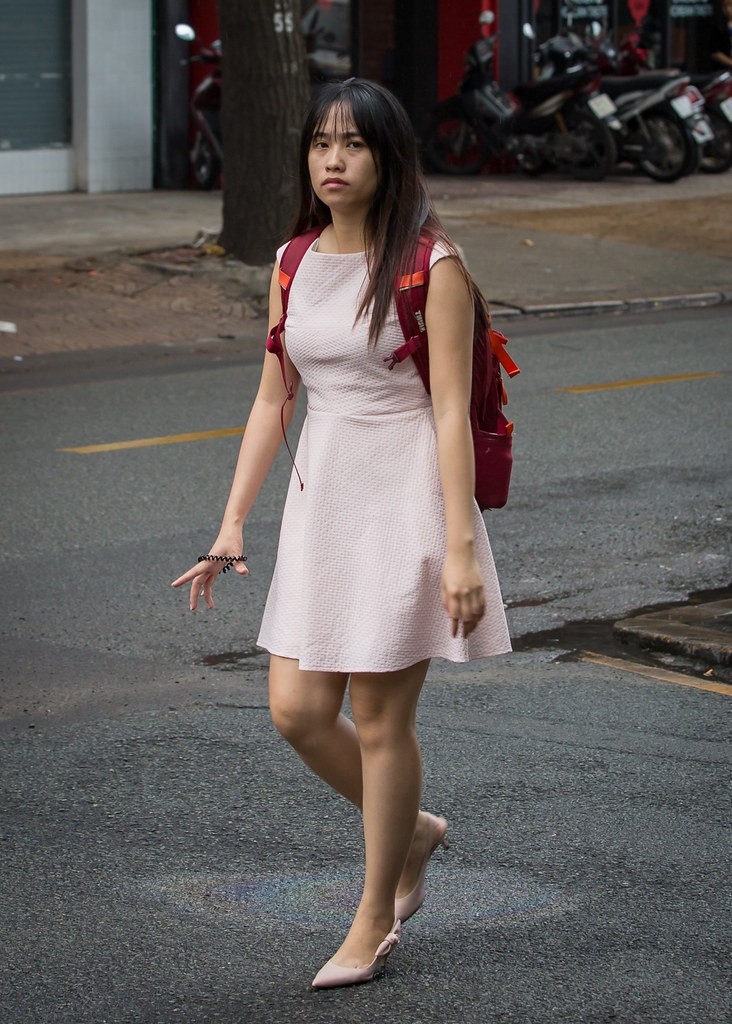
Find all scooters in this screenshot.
[172,22,223,190]
[300,11,352,89]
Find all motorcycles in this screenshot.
[420,10,623,182]
[597,14,732,174]
[522,12,714,185]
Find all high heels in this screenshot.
[394,811,450,923]
[311,916,402,988]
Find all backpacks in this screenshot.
[266,228,518,513]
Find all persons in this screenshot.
[168,76,514,989]
